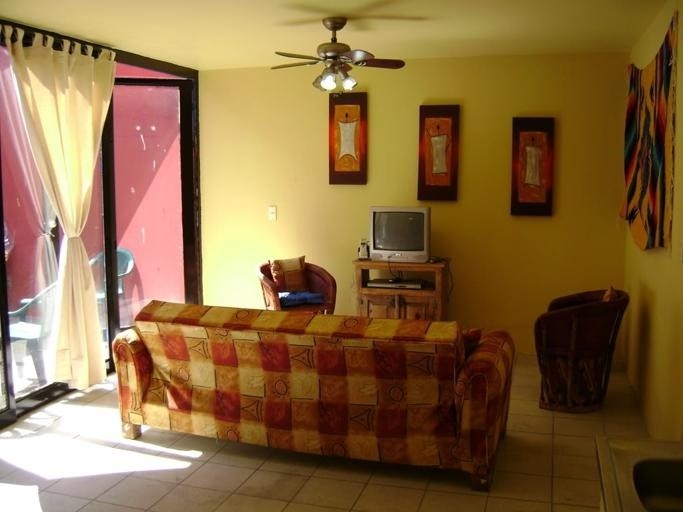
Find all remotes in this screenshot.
[429,258,441,263]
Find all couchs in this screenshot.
[110,299,515,493]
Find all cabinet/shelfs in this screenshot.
[351,258,451,321]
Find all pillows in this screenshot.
[266,255,310,292]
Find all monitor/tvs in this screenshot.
[369,207,429,262]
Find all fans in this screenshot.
[269,16,406,92]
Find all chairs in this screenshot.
[532,288,630,415]
[6,281,60,386]
[87,246,135,330]
[257,261,337,315]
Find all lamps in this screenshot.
[319,59,357,91]
